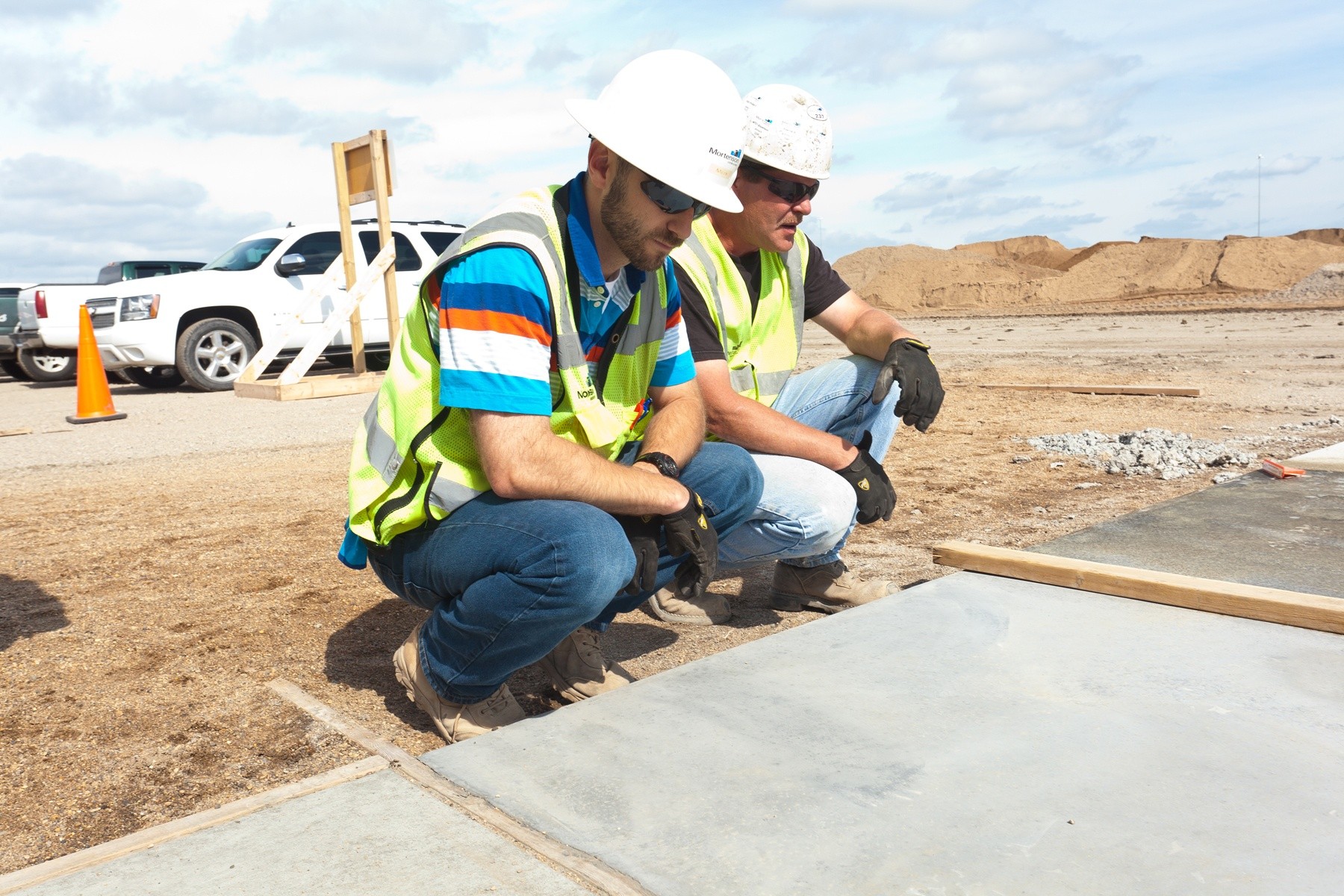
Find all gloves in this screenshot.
[872,337,947,434]
[613,515,669,596]
[834,430,895,525]
[663,485,720,598]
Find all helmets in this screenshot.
[572,50,746,214]
[744,84,833,182]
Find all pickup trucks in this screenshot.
[9,282,137,383]
[0,260,207,382]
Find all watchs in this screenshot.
[633,452,683,480]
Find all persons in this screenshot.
[641,84,946,624]
[346,48,748,743]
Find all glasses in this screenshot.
[641,175,711,220]
[762,172,821,202]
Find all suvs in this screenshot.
[84,217,470,392]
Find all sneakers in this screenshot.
[536,625,635,703]
[769,561,901,614]
[393,619,533,746]
[650,581,731,623]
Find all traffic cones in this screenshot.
[66,304,128,425]
